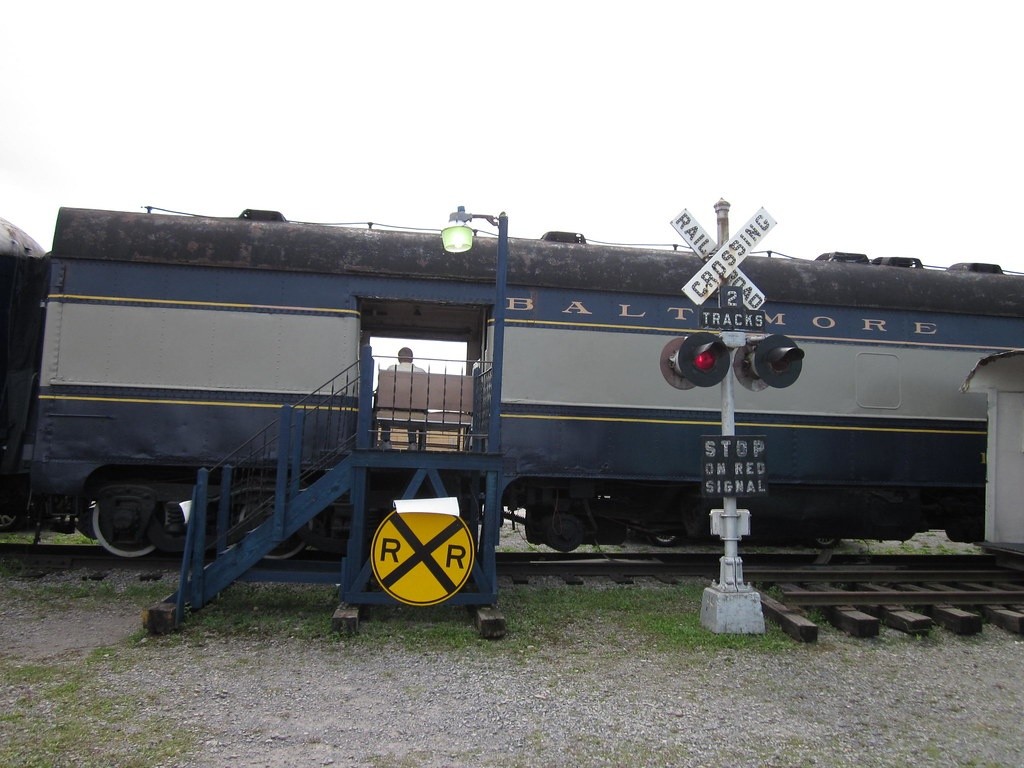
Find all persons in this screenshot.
[380,347,426,450]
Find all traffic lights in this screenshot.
[677,332,731,388]
[753,332,806,388]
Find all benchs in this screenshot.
[379,369,474,449]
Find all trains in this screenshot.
[1,201,1024,564]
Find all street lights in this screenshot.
[440,203,512,595]
